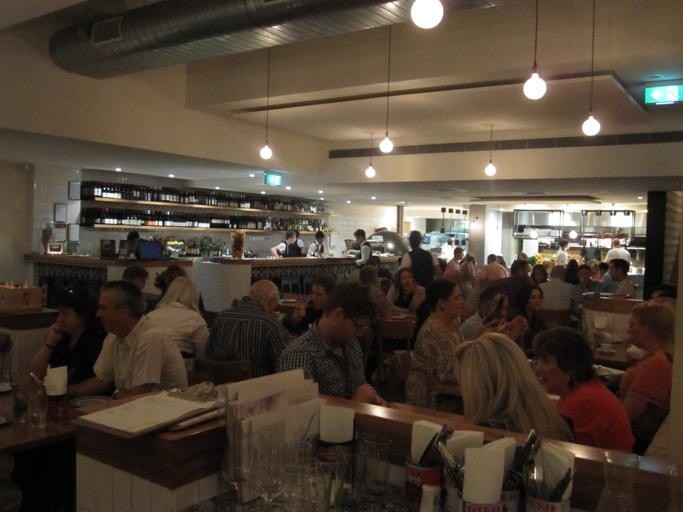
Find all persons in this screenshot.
[205,232,678,454]
[30,264,211,400]
[127,233,140,248]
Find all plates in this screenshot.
[81,195,330,234]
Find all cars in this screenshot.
[221,428,395,511]
[26,388,47,428]
[445,469,570,511]
[8,368,33,423]
[401,460,441,512]
[49,405,67,424]
[601,343,612,349]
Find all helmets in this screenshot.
[0,381,11,392]
[597,348,616,354]
[70,395,112,407]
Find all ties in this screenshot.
[137,240,162,260]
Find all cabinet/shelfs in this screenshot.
[81,195,330,234]
[184,235,226,257]
[594,447,642,512]
[47,345,71,403]
[80,181,322,231]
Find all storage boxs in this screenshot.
[0,281,44,318]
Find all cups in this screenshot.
[345,312,372,339]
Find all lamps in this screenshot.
[577,207,636,259]
[579,1,603,136]
[521,1,548,100]
[363,131,376,179]
[258,47,273,159]
[484,124,496,177]
[378,26,394,154]
[438,206,469,247]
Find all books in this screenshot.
[0,281,44,318]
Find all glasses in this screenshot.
[579,1,603,136]
[378,26,394,154]
[577,207,636,259]
[438,206,469,247]
[484,124,496,177]
[258,47,273,159]
[363,131,376,179]
[521,1,548,100]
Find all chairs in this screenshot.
[186,276,647,449]
